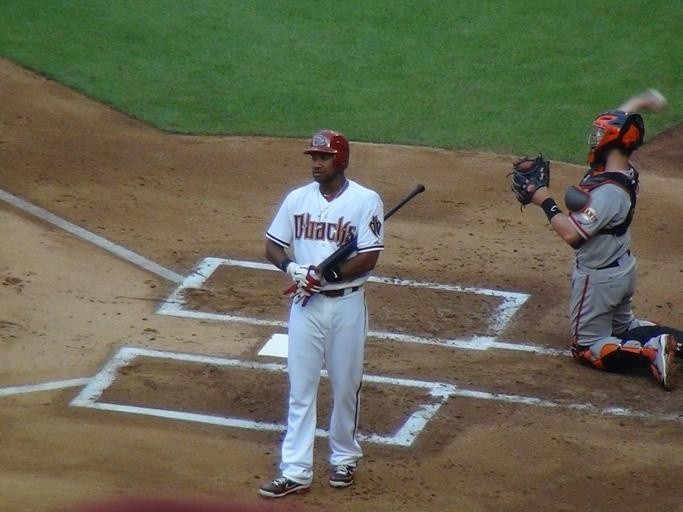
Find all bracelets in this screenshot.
[541,199,561,223]
[279,259,292,271]
[324,267,342,282]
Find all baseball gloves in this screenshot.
[506,152,550,213]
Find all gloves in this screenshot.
[285,262,321,296]
[289,273,326,307]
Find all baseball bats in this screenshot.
[315,183,425,273]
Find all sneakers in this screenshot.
[329,460,359,487]
[258,476,312,497]
[650,334,677,391]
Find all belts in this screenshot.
[319,287,358,297]
[597,249,630,270]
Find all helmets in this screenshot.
[303,130,350,172]
[586,110,644,171]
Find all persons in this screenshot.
[259,130,386,498]
[512,89,683,391]
